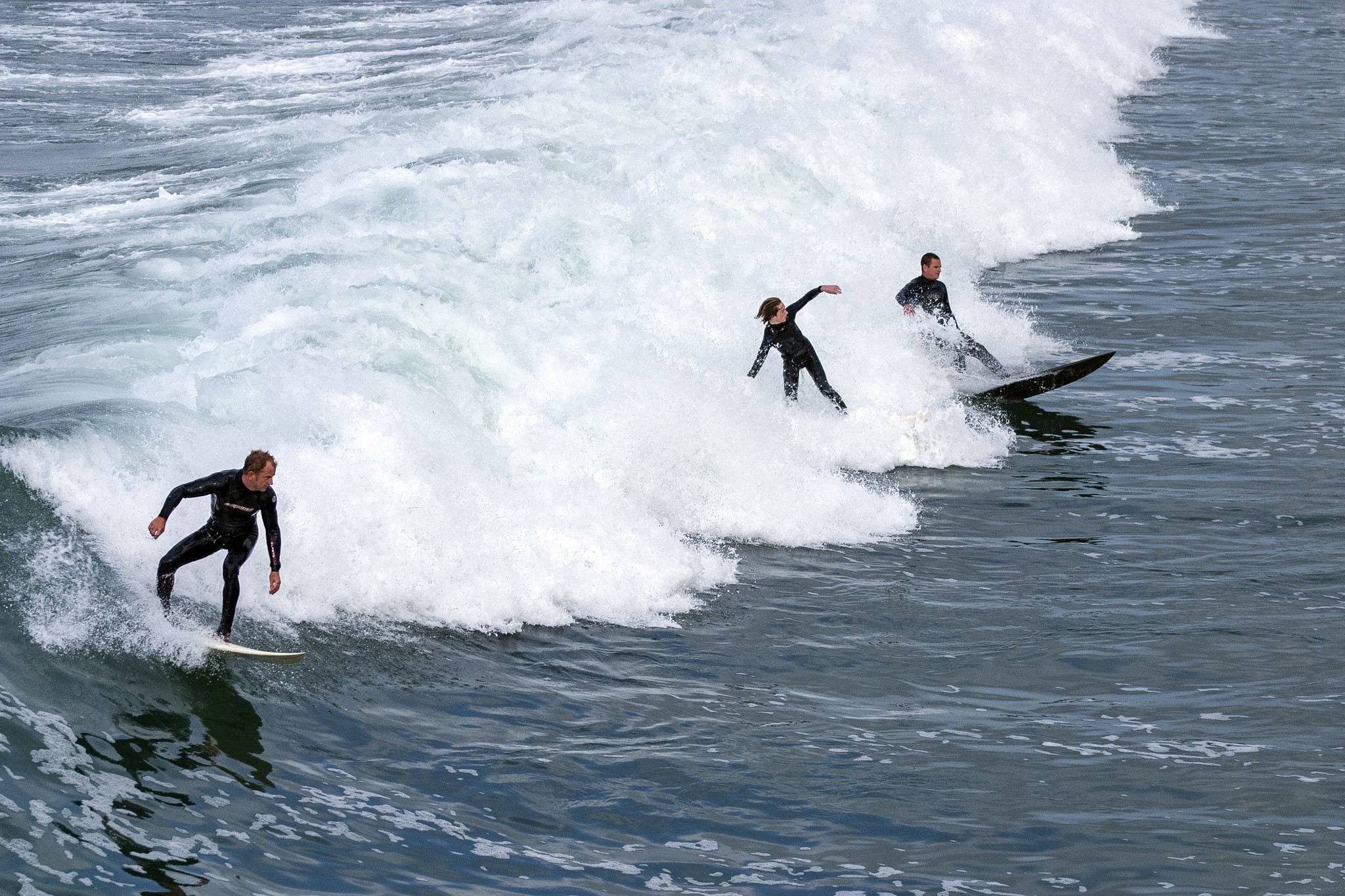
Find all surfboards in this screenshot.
[954,350,1117,406]
[208,634,307,667]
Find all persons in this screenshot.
[148,448,281,644]
[895,252,1028,379]
[747,285,847,411]
